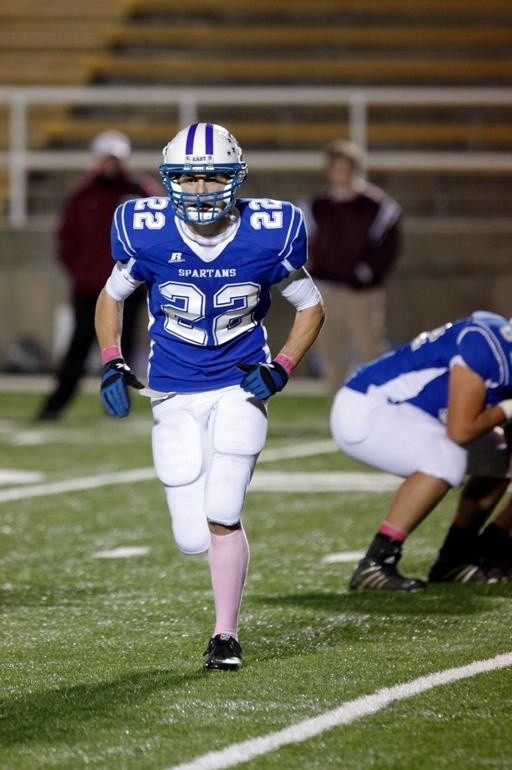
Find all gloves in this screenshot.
[99,357,145,418]
[235,360,290,401]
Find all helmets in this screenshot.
[159,121,248,226]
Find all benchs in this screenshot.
[0,0,509,150]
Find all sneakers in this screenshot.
[429,548,510,586]
[349,554,425,593]
[203,634,243,672]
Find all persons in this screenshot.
[92,120,327,678]
[298,135,404,398]
[35,128,154,424]
[327,308,512,599]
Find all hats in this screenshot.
[90,129,132,161]
